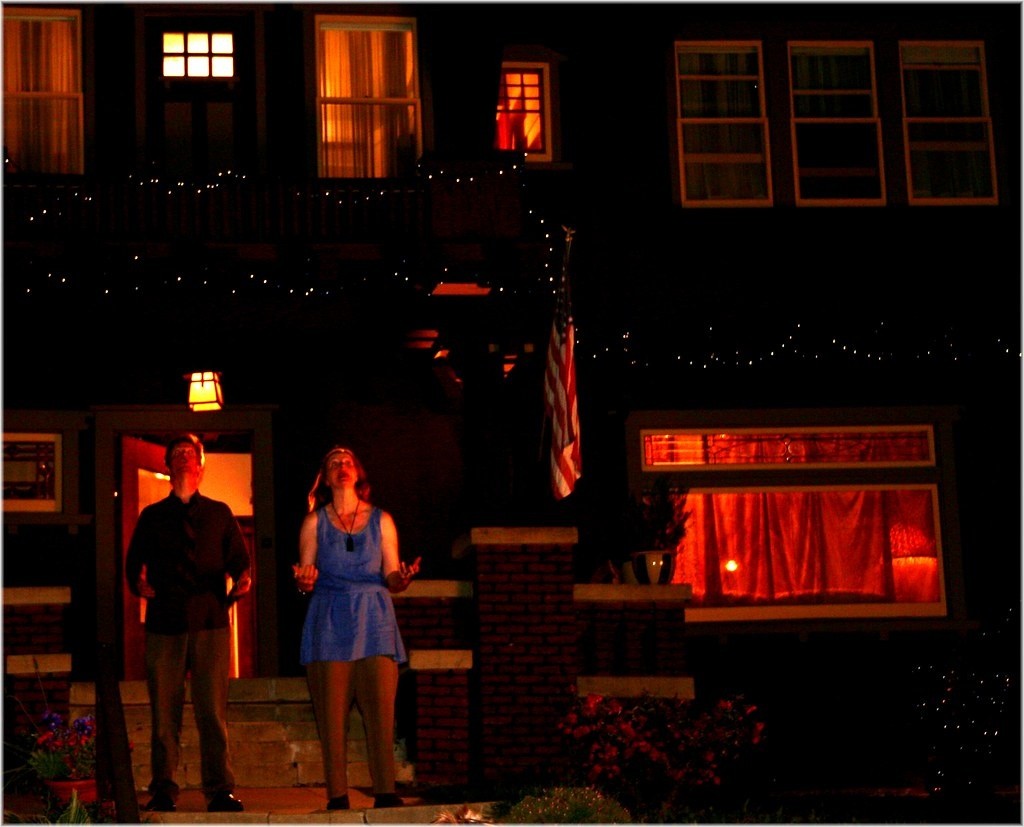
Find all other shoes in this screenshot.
[327,794,350,810]
[207,792,244,812]
[145,790,177,812]
[373,793,403,808]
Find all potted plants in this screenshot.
[593,469,692,586]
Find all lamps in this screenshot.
[182,353,224,412]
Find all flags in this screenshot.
[545,259,583,499]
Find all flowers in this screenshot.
[5,656,96,781]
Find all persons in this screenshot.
[292,446,422,810]
[126,437,252,812]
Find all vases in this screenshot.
[42,778,99,807]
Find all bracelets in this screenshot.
[298,585,306,595]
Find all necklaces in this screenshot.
[332,498,360,552]
